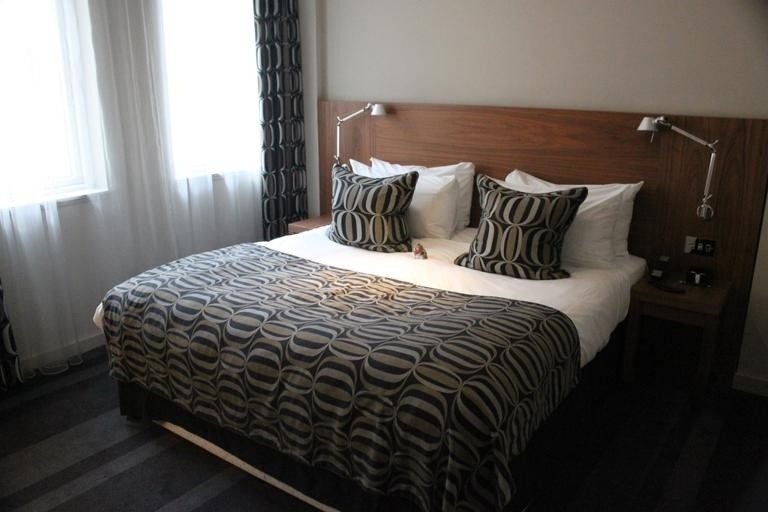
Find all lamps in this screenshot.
[336,103,386,159]
[636,118,717,220]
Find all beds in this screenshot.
[93,228,644,512]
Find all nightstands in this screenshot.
[288,215,331,234]
[624,277,731,397]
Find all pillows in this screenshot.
[485,176,630,268]
[326,163,419,252]
[454,174,588,280]
[349,158,458,240]
[505,169,643,262]
[370,157,474,230]
